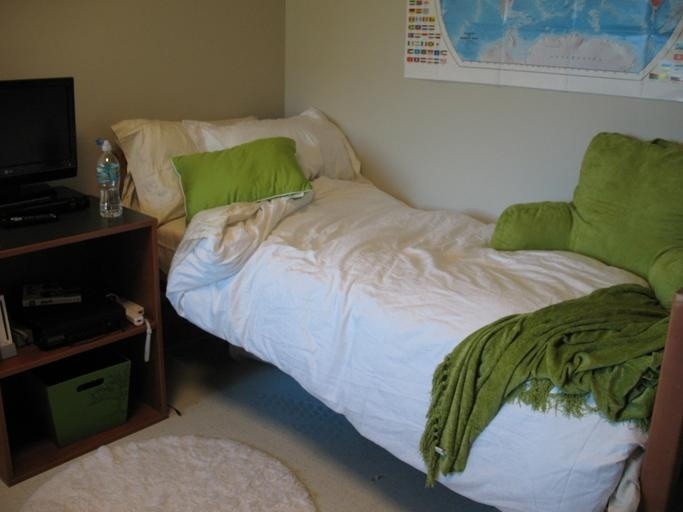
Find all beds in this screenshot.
[109,145,680,511]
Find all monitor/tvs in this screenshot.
[0,77,90,227]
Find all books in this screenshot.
[19,280,82,306]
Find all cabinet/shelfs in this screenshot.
[0,185,169,487]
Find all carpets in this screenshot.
[21,435,318,512]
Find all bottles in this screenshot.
[95,140,124,219]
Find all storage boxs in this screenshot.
[32,350,131,449]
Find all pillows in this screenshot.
[172,136,313,228]
[489,131,681,315]
[179,106,357,182]
[111,110,259,228]
